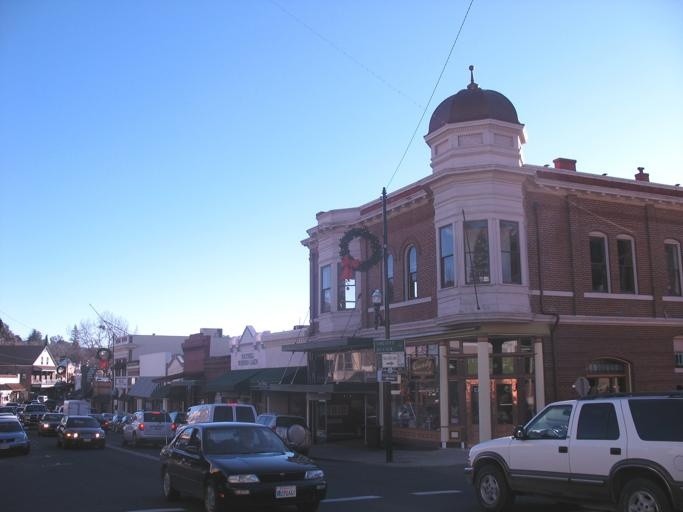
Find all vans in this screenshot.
[185,404,261,425]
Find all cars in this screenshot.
[56,415,106,449]
[122,410,177,449]
[0,395,131,433]
[159,421,328,512]
[0,415,31,456]
[168,411,187,427]
[37,412,63,436]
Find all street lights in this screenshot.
[371,187,393,465]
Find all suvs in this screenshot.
[467,391,683,512]
[258,415,313,456]
